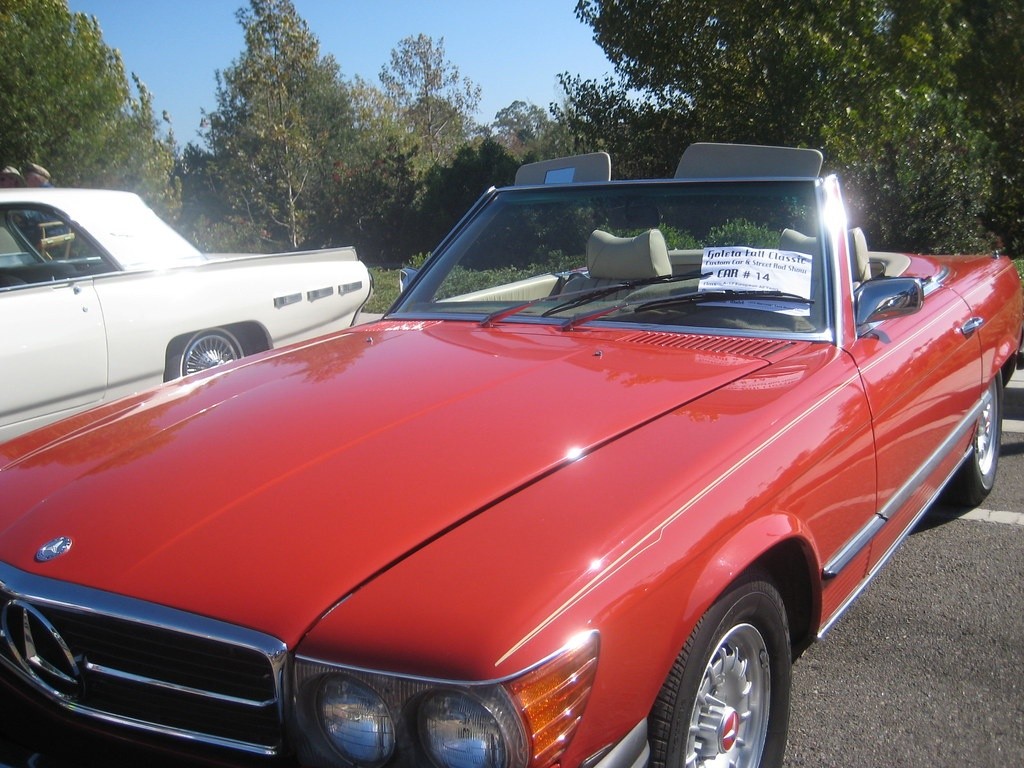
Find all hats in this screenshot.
[24,162,51,180]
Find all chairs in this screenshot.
[777,228,872,325]
[554,230,672,303]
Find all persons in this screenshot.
[0,162,73,254]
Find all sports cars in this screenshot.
[0,145,1024,768]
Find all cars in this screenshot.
[0,188,374,447]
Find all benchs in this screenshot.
[668,247,910,278]
